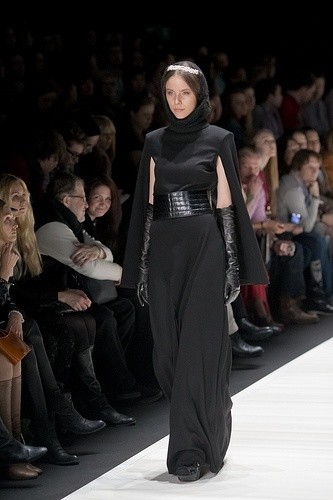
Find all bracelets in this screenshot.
[261,221,264,229]
[312,195,319,199]
[92,244,101,254]
[247,192,256,201]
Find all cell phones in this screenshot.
[290,213,301,225]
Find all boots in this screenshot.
[280,295,319,323]
[53,393,106,434]
[42,433,79,465]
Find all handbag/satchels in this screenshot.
[72,272,118,306]
[0,330,32,365]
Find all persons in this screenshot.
[0,28,333,480]
[135,61,270,482]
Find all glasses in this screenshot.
[69,195,88,202]
[68,150,81,158]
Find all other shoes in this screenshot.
[109,383,162,403]
[308,300,333,316]
[178,464,201,481]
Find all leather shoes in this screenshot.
[239,318,284,340]
[7,462,43,480]
[229,331,264,358]
[0,438,48,464]
[96,405,136,426]
